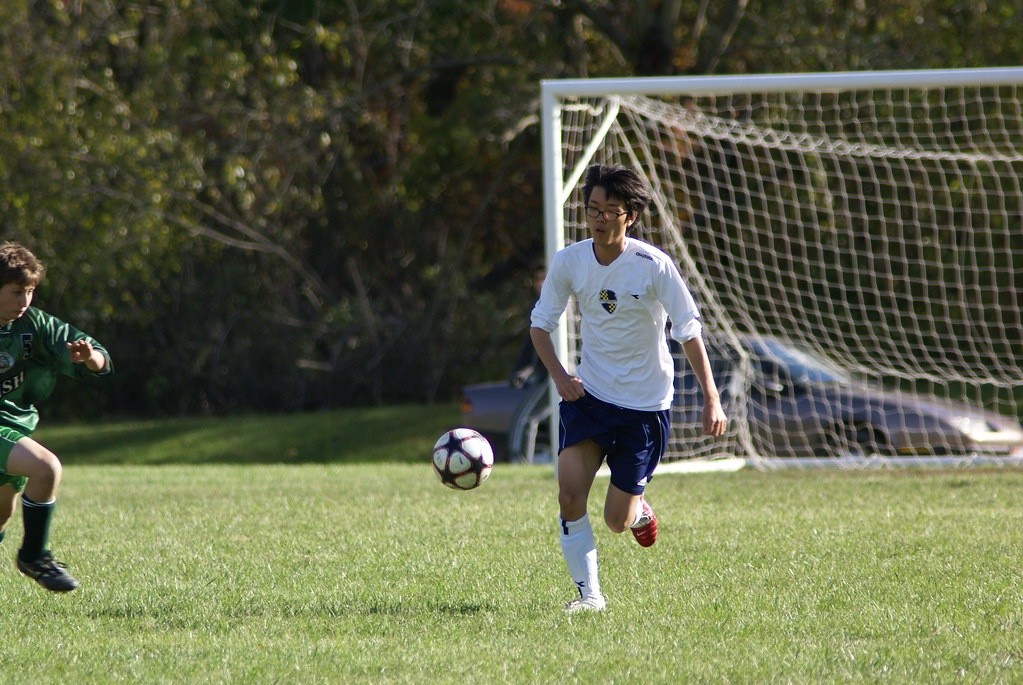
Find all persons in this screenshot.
[0,241,115,595]
[530,165,727,614]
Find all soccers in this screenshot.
[430,426,496,493]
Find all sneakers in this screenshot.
[17,550,78,591]
[630,494,658,547]
[564,597,605,616]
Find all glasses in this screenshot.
[584,206,629,222]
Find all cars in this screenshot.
[458,328,1022,459]
